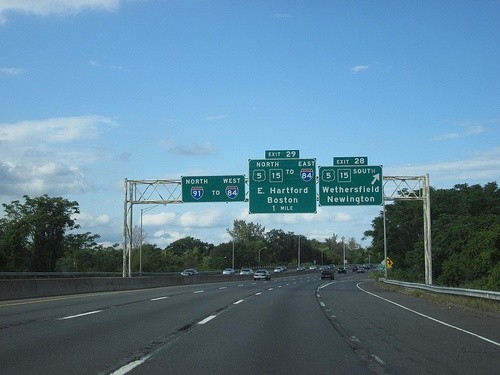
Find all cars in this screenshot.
[239,268,254,276]
[222,268,235,276]
[274,264,371,280]
[181,269,199,277]
[253,270,271,281]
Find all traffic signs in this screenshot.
[317,165,384,207]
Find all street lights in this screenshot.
[140,204,167,278]
[321,247,329,265]
[232,225,250,269]
[258,246,268,266]
